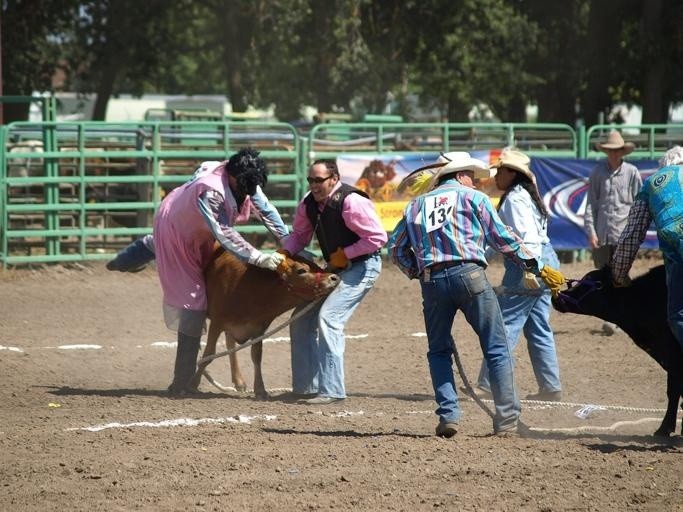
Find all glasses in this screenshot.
[307,176,332,183]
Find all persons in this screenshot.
[275,157,388,403]
[459,150,562,403]
[613,145,683,346]
[106,146,318,394]
[386,151,567,439]
[584,129,642,332]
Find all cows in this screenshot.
[184,237,341,399]
[550,259,682,438]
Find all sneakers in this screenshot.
[460,382,493,400]
[492,419,537,437]
[434,421,460,438]
[525,389,563,403]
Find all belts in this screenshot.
[429,261,456,272]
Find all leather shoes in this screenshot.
[168,379,203,396]
[105,255,149,273]
[279,390,316,398]
[296,391,343,405]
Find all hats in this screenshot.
[431,150,491,185]
[595,129,634,155]
[490,150,537,182]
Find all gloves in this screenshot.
[519,268,538,291]
[247,247,286,273]
[300,248,316,262]
[330,247,346,271]
[539,264,565,297]
